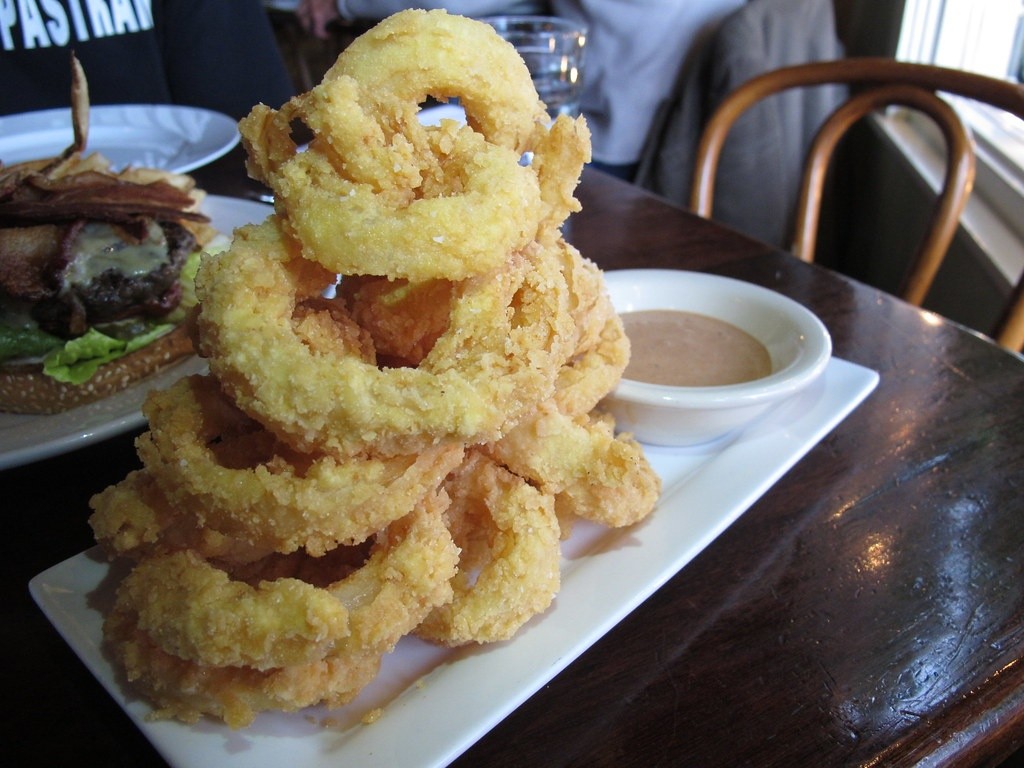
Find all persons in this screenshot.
[1,0,754,181]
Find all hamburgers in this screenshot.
[0,152,227,415]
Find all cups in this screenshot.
[476,14,588,120]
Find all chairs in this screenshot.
[691,59,1023,362]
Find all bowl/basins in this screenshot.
[573,267,833,448]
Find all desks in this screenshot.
[0,168,1024,768]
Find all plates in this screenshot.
[0,193,341,471]
[0,104,244,176]
[28,354,880,768]
